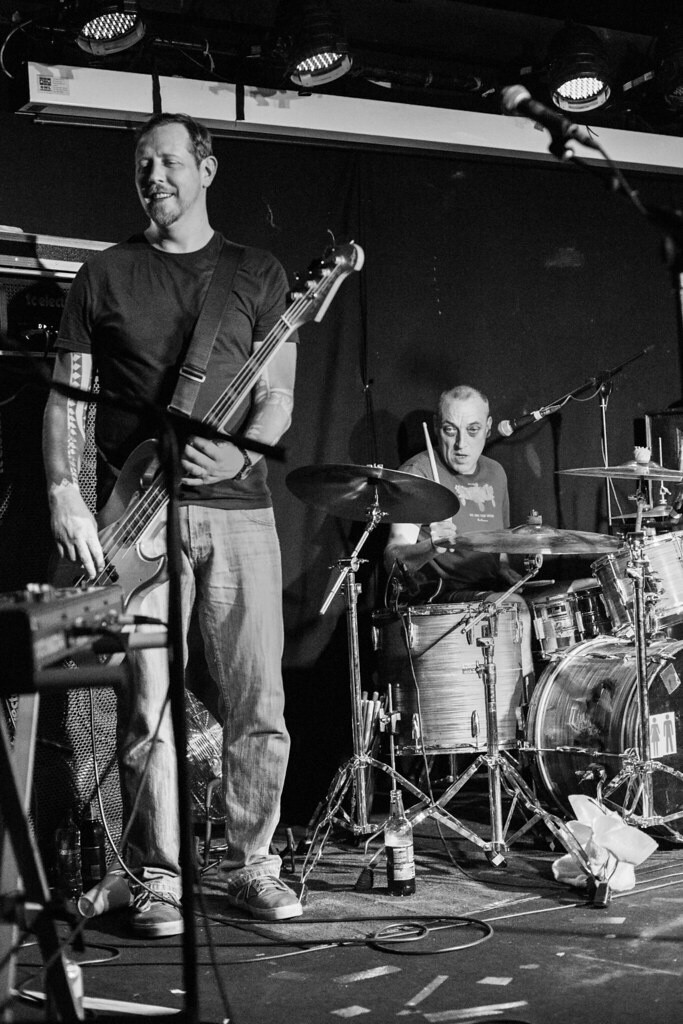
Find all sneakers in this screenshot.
[229,873,302,920]
[128,891,184,937]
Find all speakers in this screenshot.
[0,649,142,907]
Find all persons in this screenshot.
[41,113,304,936]
[389,385,544,710]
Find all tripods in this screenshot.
[295,478,683,907]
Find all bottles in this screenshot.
[82,800,107,889]
[385,790,415,895]
[56,808,83,894]
[58,921,84,1020]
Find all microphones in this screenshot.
[500,84,600,149]
[395,557,419,596]
[498,405,562,436]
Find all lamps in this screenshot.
[280,14,354,87]
[547,26,616,115]
[68,0,147,55]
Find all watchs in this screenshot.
[233,447,252,481]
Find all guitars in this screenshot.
[46,237,365,620]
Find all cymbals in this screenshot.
[432,525,626,555]
[284,462,461,525]
[553,460,683,482]
[611,504,673,519]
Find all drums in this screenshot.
[530,584,617,662]
[527,634,683,843]
[371,599,524,756]
[598,531,682,638]
